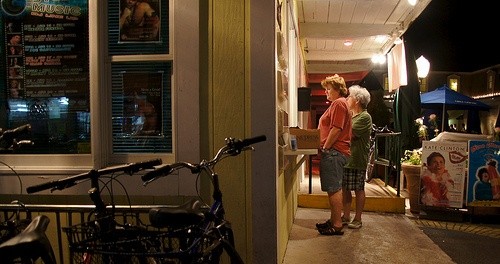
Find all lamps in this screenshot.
[392,30,402,45]
[343,39,352,46]
[416,55,430,85]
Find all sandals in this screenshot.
[316,220,333,228]
[320,225,344,235]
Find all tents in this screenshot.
[420,86,492,132]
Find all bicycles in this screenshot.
[364,125,391,182]
[0,134,269,264]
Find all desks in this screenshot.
[0,193,196,264]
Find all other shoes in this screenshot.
[341,216,352,224]
[348,219,362,229]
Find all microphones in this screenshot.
[443,170,454,185]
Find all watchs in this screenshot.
[322,146,328,150]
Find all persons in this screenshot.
[10,58,19,66]
[6,23,20,33]
[422,152,454,207]
[8,68,18,77]
[495,109,500,142]
[340,85,372,229]
[8,35,21,44]
[10,89,22,99]
[315,75,352,236]
[134,90,156,137]
[462,123,465,130]
[9,80,19,88]
[8,46,16,55]
[428,114,439,139]
[120,0,160,39]
[450,124,457,130]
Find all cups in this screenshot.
[283,126,289,144]
[290,135,297,150]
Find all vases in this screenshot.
[401,164,445,215]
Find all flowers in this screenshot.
[400,147,422,166]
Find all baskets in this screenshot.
[62,214,203,264]
